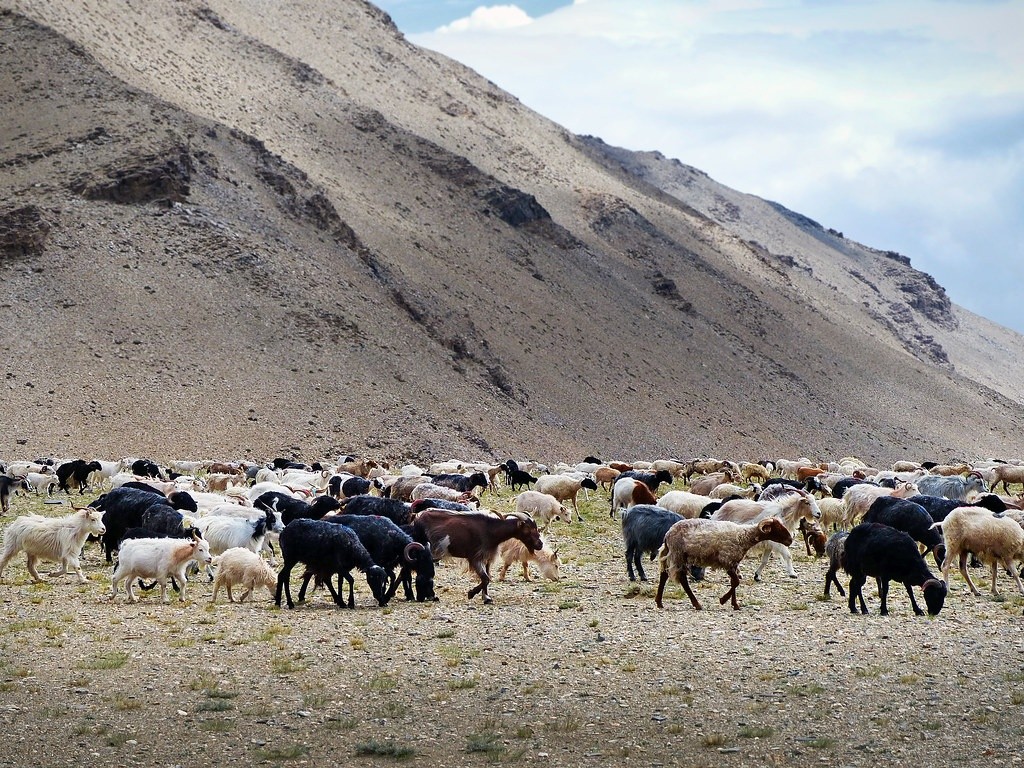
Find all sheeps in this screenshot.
[0,455,1024,620]
